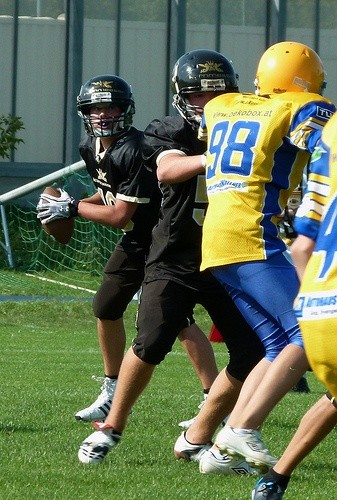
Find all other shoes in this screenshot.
[291,376,312,393]
[178,394,226,429]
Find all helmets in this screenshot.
[172,49,240,128]
[75,74,135,138]
[255,41,326,97]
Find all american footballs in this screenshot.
[38,186,75,244]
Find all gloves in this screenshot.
[35,186,81,225]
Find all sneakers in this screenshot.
[75,378,117,421]
[174,430,214,461]
[251,473,289,500]
[199,449,259,476]
[215,425,277,467]
[77,425,123,465]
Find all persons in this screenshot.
[35,74,222,428]
[79,42,337,500]
